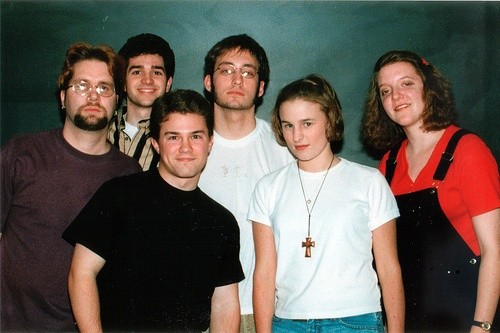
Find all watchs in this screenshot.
[471,320,492,331]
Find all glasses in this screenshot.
[214,64,259,79]
[65,80,117,97]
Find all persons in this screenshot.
[107,33,176,173]
[0,42,144,333]
[360,50,500,333]
[246,73,407,333]
[61,90,245,333]
[156,35,299,333]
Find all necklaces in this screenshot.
[297,153,334,257]
